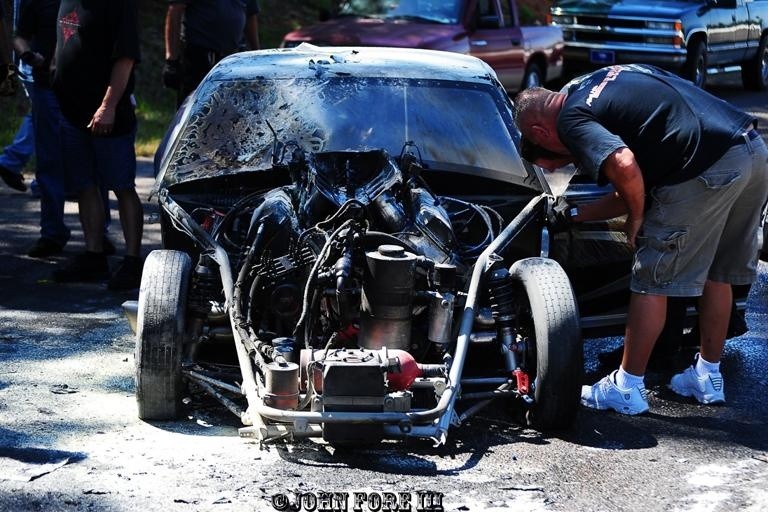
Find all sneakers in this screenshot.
[580,369,649,415]
[29,238,142,290]
[671,365,725,404]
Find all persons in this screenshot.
[163,0,259,115]
[514,64,766,416]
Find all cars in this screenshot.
[131,44,755,455]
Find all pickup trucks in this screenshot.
[278,1,562,95]
[550,0,768,89]
